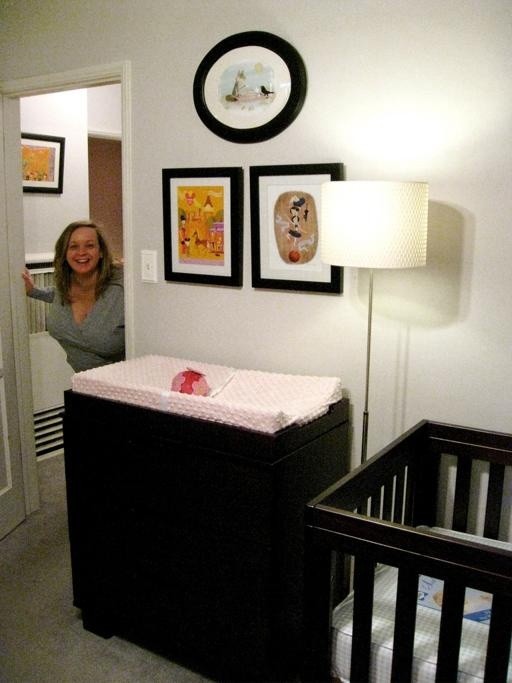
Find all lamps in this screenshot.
[316,178,430,464]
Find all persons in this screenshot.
[21,219,126,373]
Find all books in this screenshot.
[416,575,492,625]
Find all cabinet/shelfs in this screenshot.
[59,387,376,681]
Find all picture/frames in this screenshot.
[21,131,66,194]
[159,163,346,296]
[191,30,308,148]
